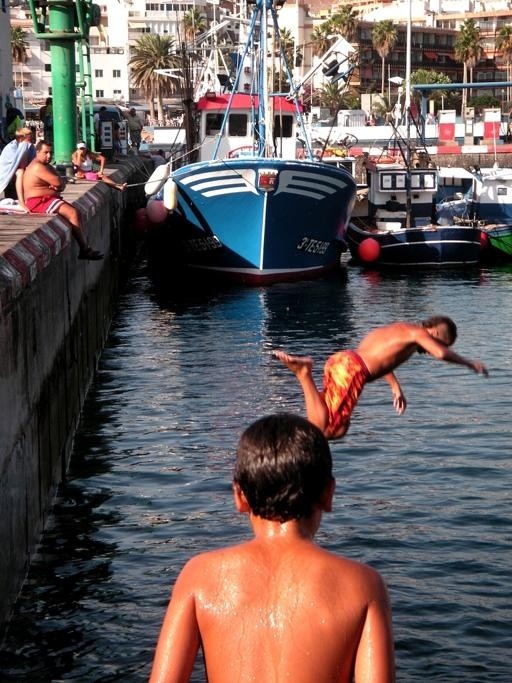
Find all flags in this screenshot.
[438,108,456,140]
[483,107,501,138]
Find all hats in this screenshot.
[77,142,86,148]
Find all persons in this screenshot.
[121,107,143,150]
[39,97,53,142]
[272,316,490,442]
[6,102,24,126]
[94,106,107,151]
[22,137,105,261]
[0,126,38,207]
[111,117,123,161]
[71,138,127,191]
[148,414,397,682]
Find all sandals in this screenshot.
[77,247,105,260]
